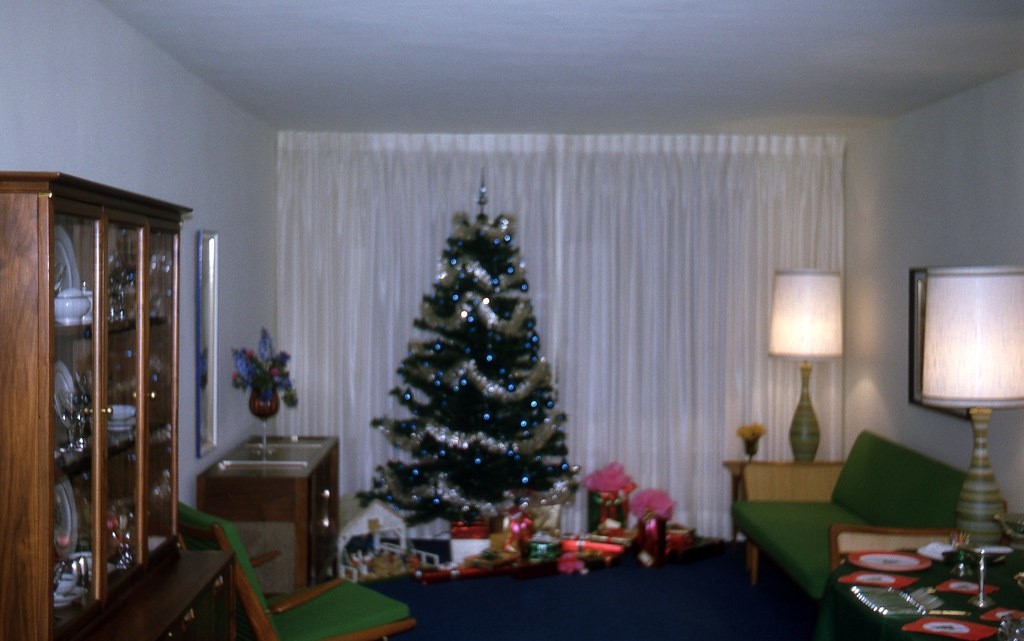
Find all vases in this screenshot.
[744,438,759,459]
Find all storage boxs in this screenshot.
[450,493,695,568]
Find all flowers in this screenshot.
[736,422,768,440]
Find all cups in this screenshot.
[54,287,91,327]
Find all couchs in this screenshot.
[733,428,964,620]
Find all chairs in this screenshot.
[181,501,414,641]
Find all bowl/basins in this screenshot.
[55,573,78,594]
[54,587,89,607]
[108,404,136,421]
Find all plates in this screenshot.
[54,225,80,293]
[847,549,932,571]
[52,474,79,556]
[54,360,75,428]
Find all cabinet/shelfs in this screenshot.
[198,435,342,593]
[0,170,246,641]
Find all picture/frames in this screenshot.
[193,230,219,456]
[907,267,975,418]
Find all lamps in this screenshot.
[919,265,1024,543]
[769,271,846,460]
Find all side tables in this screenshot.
[723,460,846,586]
[813,541,1024,641]
